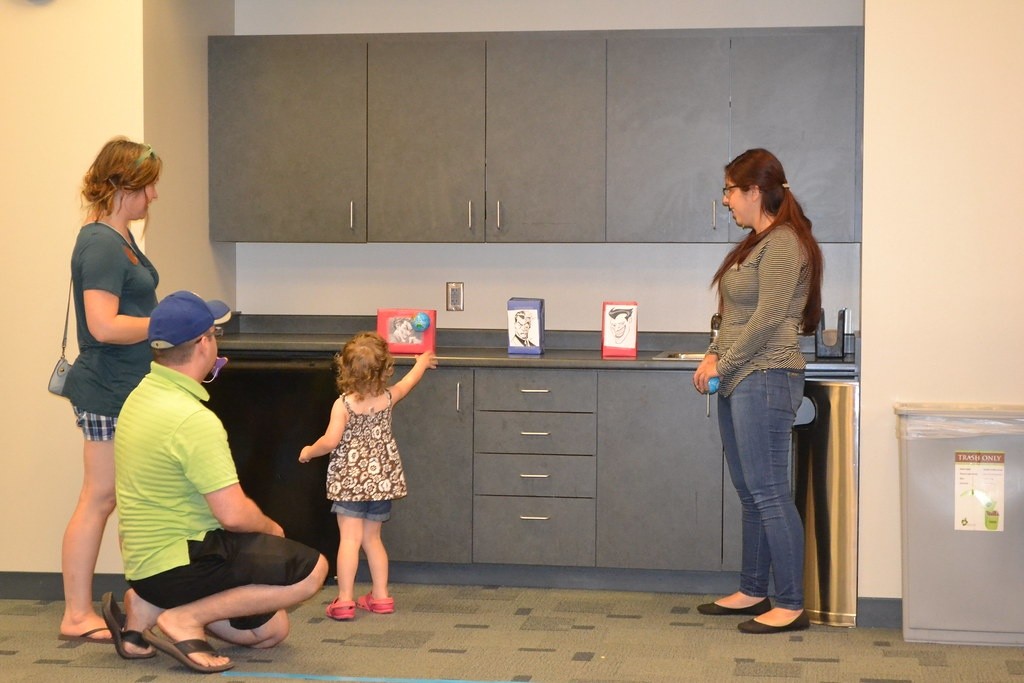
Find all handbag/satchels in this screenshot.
[48,357,73,397]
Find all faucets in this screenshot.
[710,312,722,344]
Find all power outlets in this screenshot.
[447,282,464,313]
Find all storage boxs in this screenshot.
[508,297,546,353]
[601,301,639,361]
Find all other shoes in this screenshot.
[738,608,810,634]
[698,597,771,615]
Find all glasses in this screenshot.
[136,143,157,167]
[723,184,762,195]
[195,327,224,343]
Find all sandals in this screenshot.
[357,591,395,614]
[326,597,357,620]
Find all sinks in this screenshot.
[651,351,706,361]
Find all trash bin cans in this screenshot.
[893,401,1024,648]
[791,380,856,629]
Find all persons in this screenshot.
[299,330,439,621]
[103,290,328,673]
[693,149,823,633]
[58,137,162,644]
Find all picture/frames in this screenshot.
[377,306,438,355]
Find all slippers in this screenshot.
[59,628,116,644]
[102,592,156,660]
[143,629,235,671]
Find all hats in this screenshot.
[149,290,232,349]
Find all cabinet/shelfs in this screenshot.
[207,24,865,244]
[381,366,791,572]
